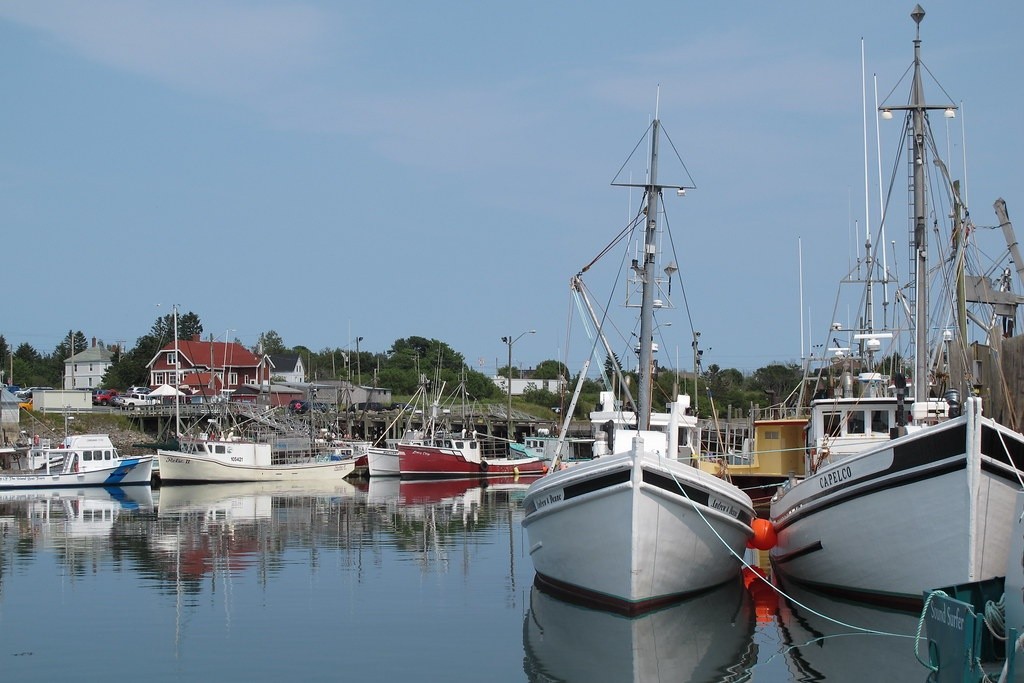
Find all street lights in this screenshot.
[501,330,536,438]
[209,329,237,389]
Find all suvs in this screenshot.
[75,387,161,410]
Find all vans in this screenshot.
[348,403,382,415]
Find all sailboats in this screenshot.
[769,3,1023,615]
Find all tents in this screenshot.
[148,384,185,404]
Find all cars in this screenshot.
[3,384,53,413]
[390,402,413,412]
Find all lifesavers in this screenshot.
[479,461,488,471]
[479,479,489,488]
[34,435,39,445]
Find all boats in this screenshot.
[0,382,153,490]
[522,63,755,613]
[693,353,878,514]
[158,478,356,669]
[157,319,595,487]
[0,484,155,593]
[367,470,544,606]
[520,575,759,683]
[773,571,944,683]
